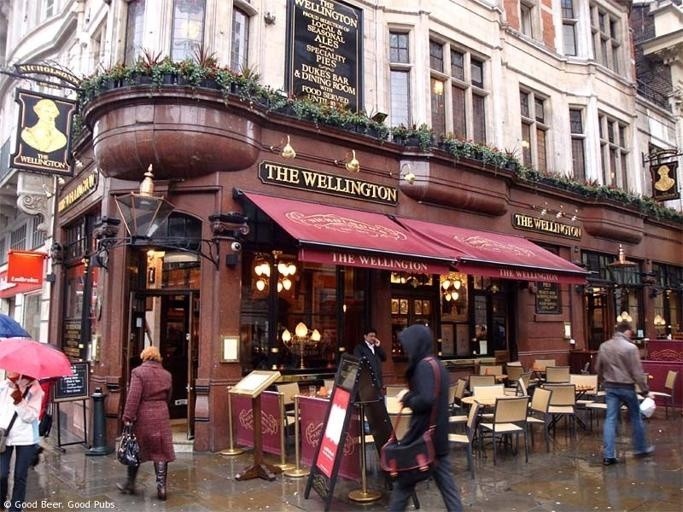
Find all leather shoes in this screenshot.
[634,447,654,459]
[603,457,619,465]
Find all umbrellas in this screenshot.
[0,313,33,337]
[0,338,73,387]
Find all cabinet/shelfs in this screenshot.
[569,347,598,375]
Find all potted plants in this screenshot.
[177,56,201,102]
[475,144,494,165]
[504,145,519,169]
[456,141,473,161]
[149,60,177,99]
[207,66,232,108]
[407,124,431,153]
[190,43,218,88]
[349,112,367,136]
[523,167,683,222]
[131,56,149,84]
[438,139,460,160]
[99,65,120,88]
[390,126,407,144]
[367,118,389,145]
[253,83,349,131]
[120,66,131,86]
[231,75,253,110]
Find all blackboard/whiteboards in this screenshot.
[55,363,87,399]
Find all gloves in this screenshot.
[11,390,22,404]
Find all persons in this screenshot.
[385,325,466,512]
[0,369,45,512]
[115,345,173,501]
[354,327,387,409]
[593,323,656,466]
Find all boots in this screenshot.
[154,461,168,501]
[116,463,140,495]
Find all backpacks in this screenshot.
[38,414,53,438]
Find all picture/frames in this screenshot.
[220,335,241,363]
[564,321,572,339]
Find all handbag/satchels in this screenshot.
[379,430,436,472]
[117,433,140,468]
[0,427,7,453]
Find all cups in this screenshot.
[309,386,316,397]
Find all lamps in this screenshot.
[255,256,296,292]
[616,311,633,323]
[653,314,666,326]
[389,164,416,185]
[440,272,464,301]
[270,134,296,161]
[334,149,360,173]
[282,322,320,368]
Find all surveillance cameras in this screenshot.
[230,242,242,252]
